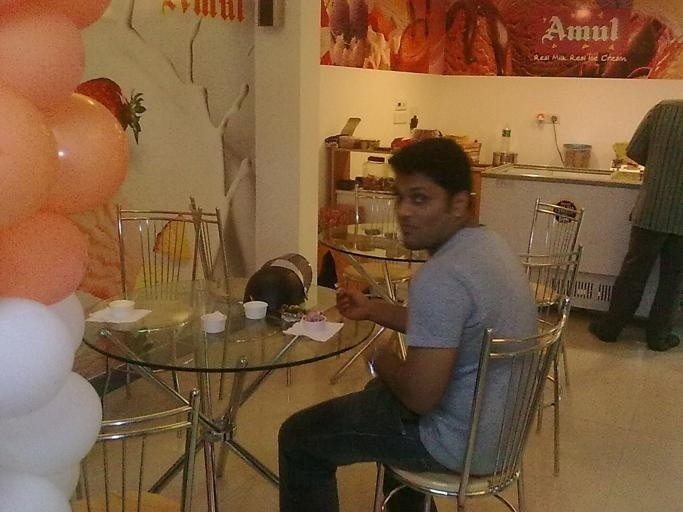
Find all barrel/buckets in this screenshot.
[563,144,593,169]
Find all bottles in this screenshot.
[491,126,518,167]
[362,156,386,191]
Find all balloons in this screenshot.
[1,0,129,511]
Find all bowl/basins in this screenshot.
[300,314,327,333]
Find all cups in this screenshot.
[199,313,227,335]
[108,298,136,319]
[242,299,269,321]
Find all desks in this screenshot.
[81,275,376,494]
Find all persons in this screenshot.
[588,100,681,349]
[275,135,541,512]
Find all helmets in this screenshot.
[244,254,312,310]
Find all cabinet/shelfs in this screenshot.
[328,143,398,205]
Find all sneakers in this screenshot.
[650,334,680,351]
[589,320,616,342]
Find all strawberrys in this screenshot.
[74,77,145,146]
